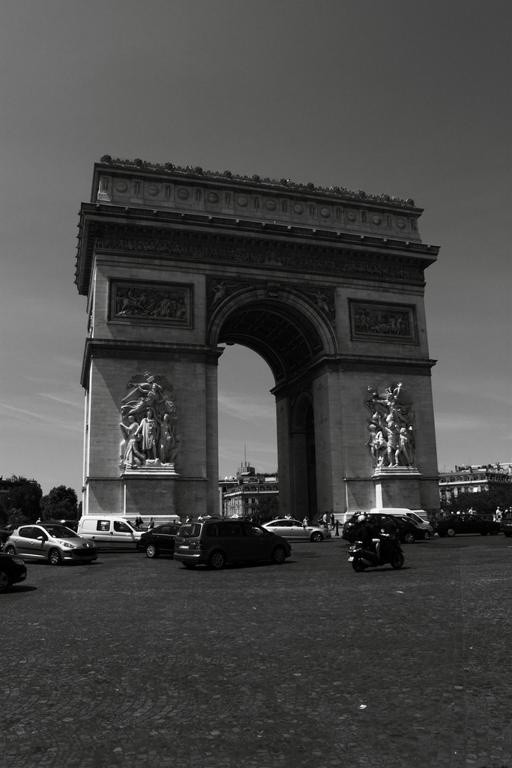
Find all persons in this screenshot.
[322,510,329,526]
[147,517,155,528]
[270,511,299,522]
[134,516,144,529]
[302,516,309,526]
[493,505,512,523]
[362,381,415,467]
[440,505,478,520]
[119,376,182,468]
[35,517,42,525]
[185,513,219,524]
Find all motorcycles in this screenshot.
[346,530,405,572]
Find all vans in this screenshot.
[76,515,144,550]
[172,520,291,567]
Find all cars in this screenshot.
[261,518,326,542]
[342,507,503,543]
[0,552,27,594]
[136,522,181,558]
[3,524,98,565]
